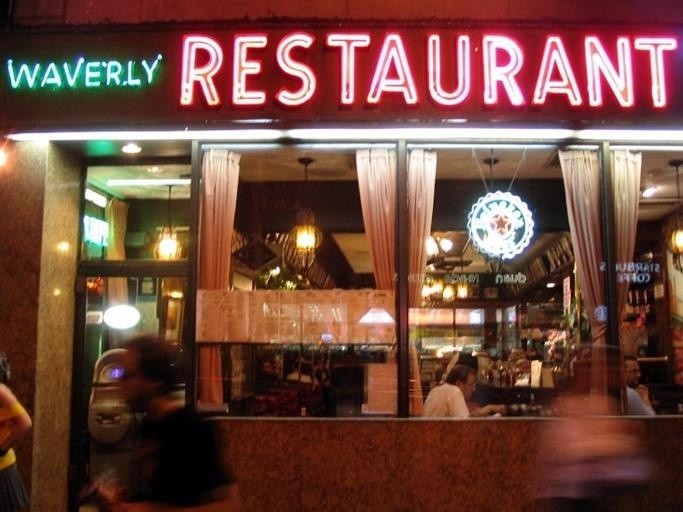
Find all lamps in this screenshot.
[667,159,683,256]
[289,157,322,253]
[640,185,657,197]
[358,308,395,324]
[467,148,535,263]
[153,185,181,259]
[422,232,470,301]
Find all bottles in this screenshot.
[628,289,653,320]
[511,393,538,417]
[141,276,154,295]
[500,362,513,386]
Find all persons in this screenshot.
[255,361,286,416]
[522,390,683,512]
[95,335,240,511]
[423,365,505,415]
[0,351,34,511]
[624,356,654,416]
[304,367,338,417]
